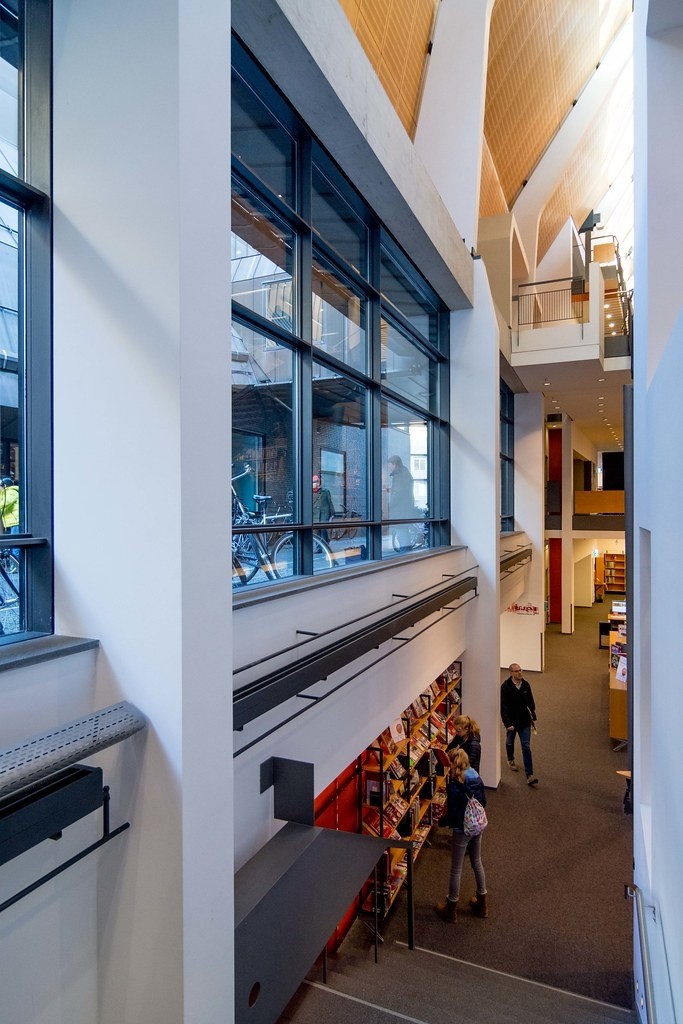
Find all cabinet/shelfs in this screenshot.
[596,553,604,603]
[609,631,631,750]
[604,554,627,592]
[358,660,463,918]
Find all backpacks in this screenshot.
[463,792,488,835]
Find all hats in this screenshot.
[313,475,320,483]
[0,478,14,486]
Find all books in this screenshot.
[615,656,628,682]
[362,662,461,910]
[606,562,615,584]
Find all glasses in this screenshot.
[511,669,523,673]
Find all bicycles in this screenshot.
[0,478,23,637]
[264,501,292,544]
[231,488,338,585]
[392,505,430,554]
[334,503,361,540]
[230,462,277,588]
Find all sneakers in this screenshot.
[527,774,538,784]
[6,565,18,573]
[507,760,518,771]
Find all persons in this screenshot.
[618,668,626,681]
[445,714,481,856]
[0,478,19,574]
[436,744,489,922]
[382,456,414,553]
[312,475,335,553]
[500,663,539,786]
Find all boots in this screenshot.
[469,890,489,918]
[436,897,458,923]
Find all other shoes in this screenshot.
[313,548,322,553]
